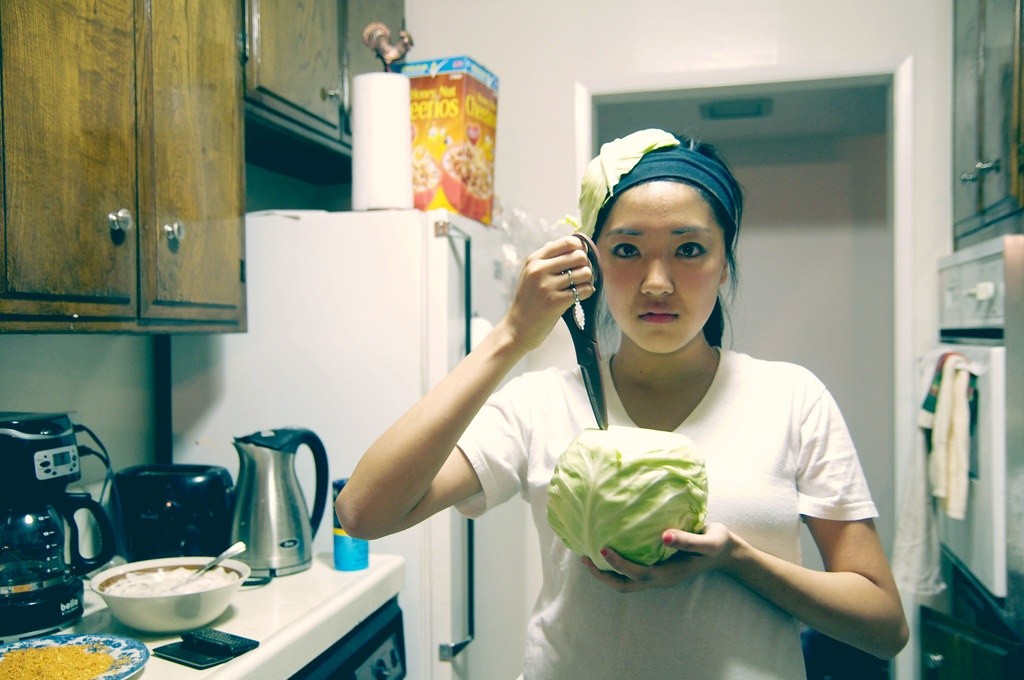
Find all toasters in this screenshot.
[108,462,234,562]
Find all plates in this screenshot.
[0,633,150,680]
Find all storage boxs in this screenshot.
[395,56,499,227]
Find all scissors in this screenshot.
[561,234,604,432]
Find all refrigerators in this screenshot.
[170,209,541,680]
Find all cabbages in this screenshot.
[564,128,680,240]
[544,424,708,575]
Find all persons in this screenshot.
[333,128,911,680]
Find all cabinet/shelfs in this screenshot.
[918,545,1024,680]
[244,0,407,185]
[953,0,1024,253]
[0,0,247,332]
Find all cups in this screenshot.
[332,478,369,572]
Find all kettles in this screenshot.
[230,427,329,587]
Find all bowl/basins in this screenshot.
[91,556,251,634]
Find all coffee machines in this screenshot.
[0,410,124,634]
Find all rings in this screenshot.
[572,288,579,302]
[567,269,573,287]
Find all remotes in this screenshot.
[180,627,259,653]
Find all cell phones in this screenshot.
[153,642,234,667]
